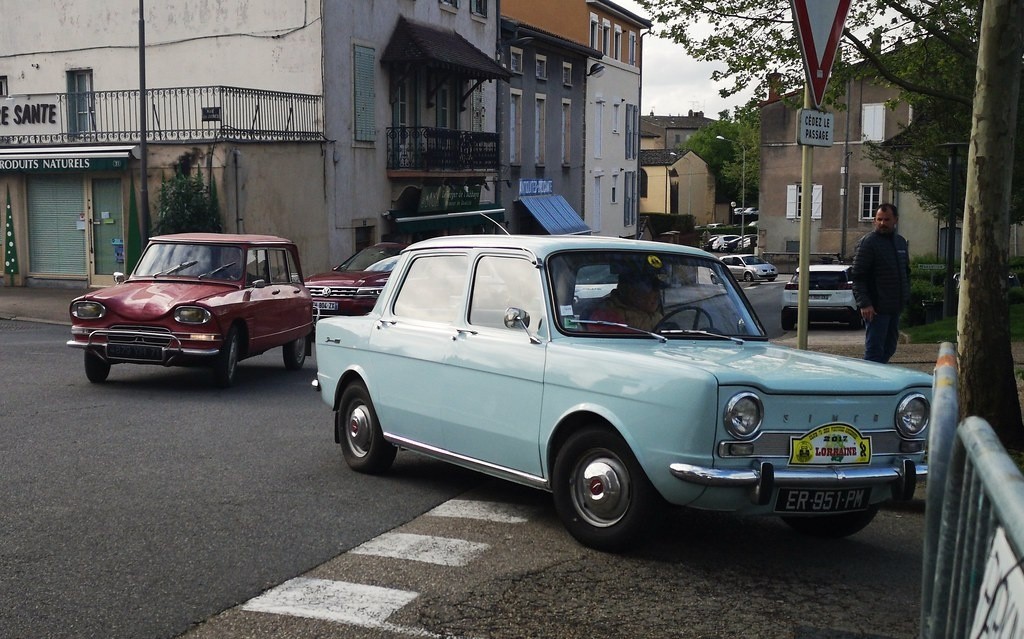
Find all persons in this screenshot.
[850,203,912,365]
[207,247,255,285]
[584,265,670,334]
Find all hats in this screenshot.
[630,272,672,289]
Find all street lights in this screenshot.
[716,135,744,235]
[670,152,692,216]
[731,201,736,226]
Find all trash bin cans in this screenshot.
[922,298,944,324]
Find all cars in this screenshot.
[728,234,751,253]
[734,207,759,215]
[66,233,314,385]
[708,254,779,284]
[748,221,759,226]
[310,235,936,546]
[303,243,410,339]
[737,236,751,249]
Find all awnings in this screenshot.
[0,145,144,174]
[502,15,606,63]
[518,193,593,235]
[379,18,517,111]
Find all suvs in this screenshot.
[707,223,725,228]
[708,235,718,251]
[712,235,741,252]
[781,264,862,330]
